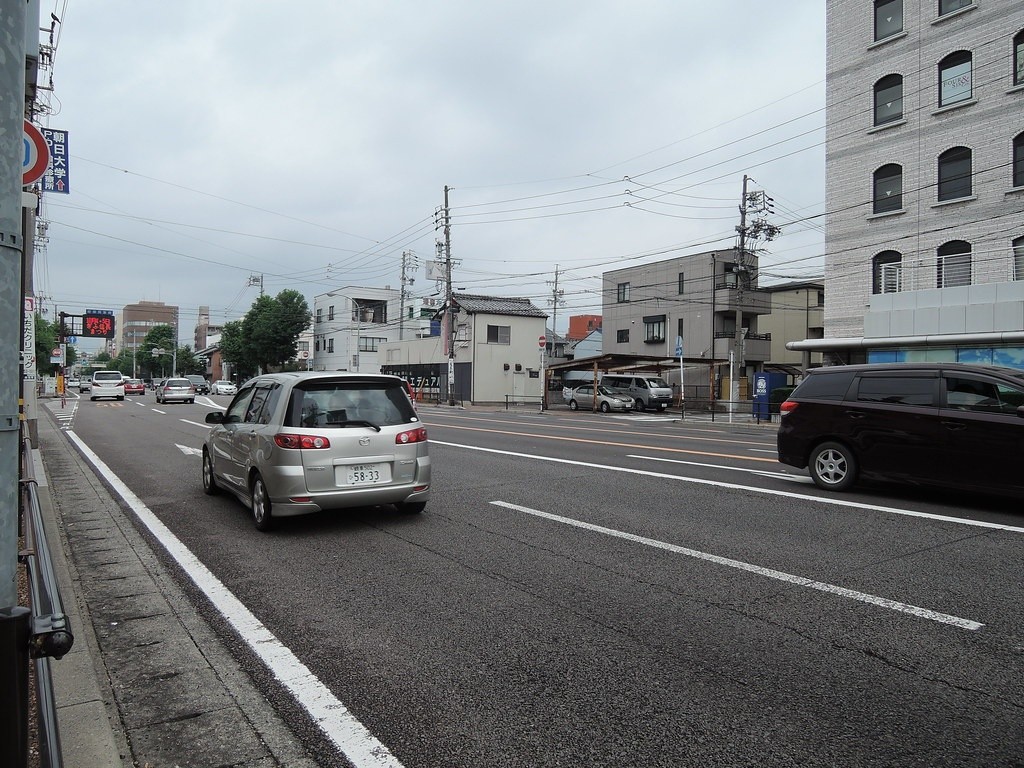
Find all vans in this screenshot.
[598,374,674,413]
[88,370,127,402]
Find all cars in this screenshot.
[211,378,238,396]
[155,378,197,405]
[68,375,147,396]
[565,383,637,415]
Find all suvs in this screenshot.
[201,370,432,533]
[775,361,1024,511]
[183,374,210,396]
